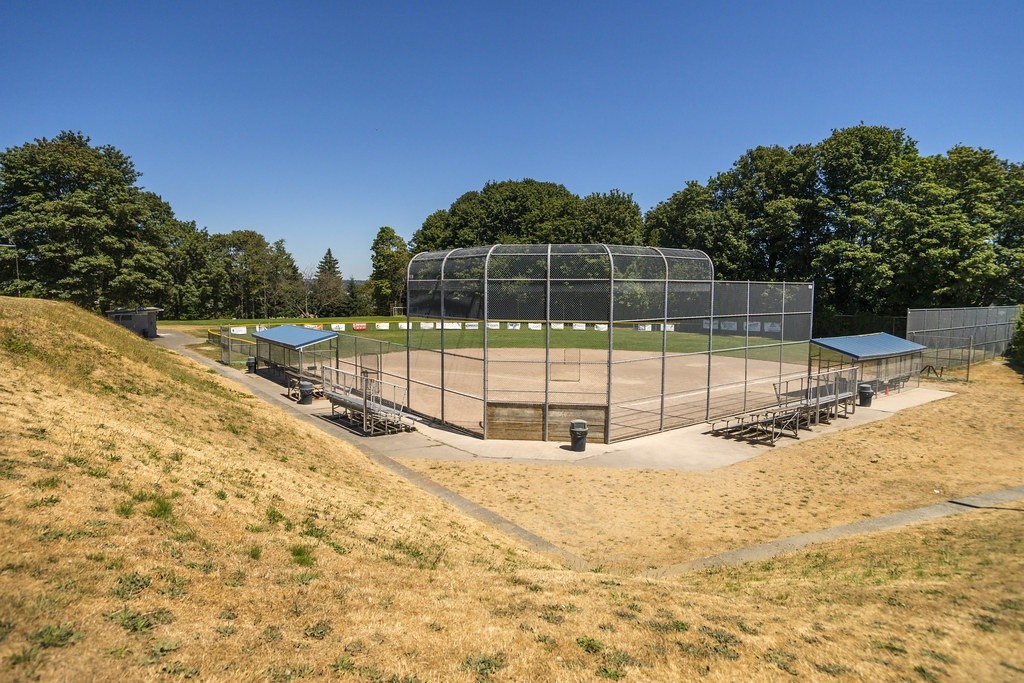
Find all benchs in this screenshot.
[708,365,860,448]
[322,364,422,438]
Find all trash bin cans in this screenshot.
[570,418,589,452]
[246,357,256,373]
[299,381,315,403]
[859,385,874,407]
